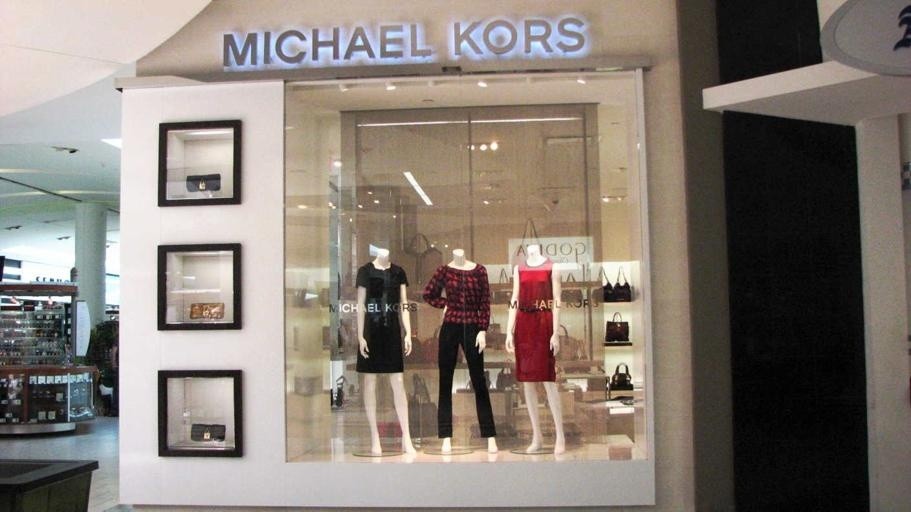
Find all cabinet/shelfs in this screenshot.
[346,280,606,372]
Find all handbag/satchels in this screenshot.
[191,424,225,441]
[408,374,438,438]
[556,325,578,360]
[190,303,224,319]
[186,174,220,191]
[561,274,583,301]
[496,359,517,389]
[588,364,633,390]
[486,324,506,349]
[403,327,462,364]
[605,313,628,341]
[490,269,513,304]
[378,422,402,451]
[592,267,631,301]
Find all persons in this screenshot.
[423,247,500,455]
[505,244,570,455]
[354,247,418,456]
